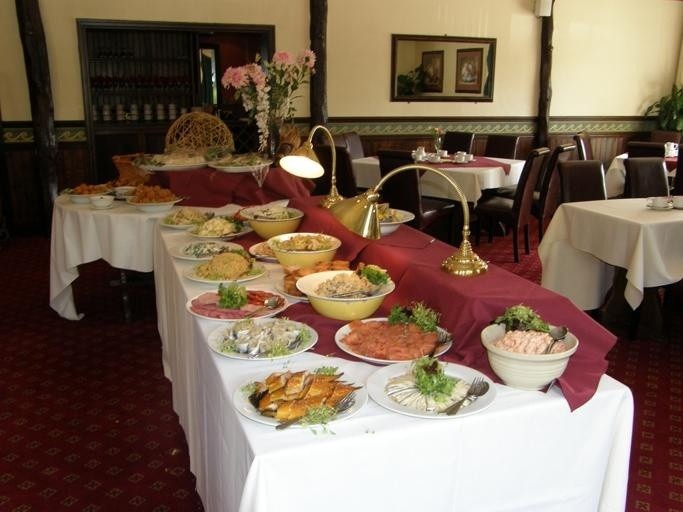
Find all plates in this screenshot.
[208,320,317,359]
[183,262,265,282]
[208,158,273,171]
[276,273,311,299]
[190,224,252,240]
[336,318,452,365]
[369,361,498,420]
[168,240,243,260]
[185,290,288,321]
[134,153,232,170]
[234,365,368,427]
[158,211,216,228]
[90,204,112,209]
[126,196,182,210]
[64,187,111,202]
[249,242,277,259]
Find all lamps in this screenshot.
[279,125,344,208]
[327,163,488,277]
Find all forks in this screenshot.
[439,375,484,415]
[429,331,448,360]
[274,393,356,432]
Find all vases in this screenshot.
[270,124,301,166]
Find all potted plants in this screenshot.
[644,83,683,144]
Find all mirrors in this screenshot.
[390,33,497,103]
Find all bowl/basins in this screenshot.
[298,270,396,320]
[240,205,304,239]
[269,233,342,268]
[379,209,415,234]
[90,196,113,206]
[480,323,579,391]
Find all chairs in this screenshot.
[531,142,577,244]
[573,130,593,160]
[676,144,683,195]
[627,141,667,158]
[376,148,455,236]
[557,159,607,203]
[623,157,671,198]
[484,133,519,160]
[441,131,475,156]
[476,147,550,263]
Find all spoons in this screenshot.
[242,297,280,323]
[543,325,570,353]
[445,381,493,418]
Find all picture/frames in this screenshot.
[418,50,445,93]
[455,48,484,94]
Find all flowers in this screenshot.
[220,47,316,154]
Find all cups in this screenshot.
[670,196,683,208]
[116,186,137,197]
[647,197,668,207]
[428,156,440,161]
[466,154,473,160]
[437,149,448,157]
[455,151,466,156]
[456,156,464,161]
